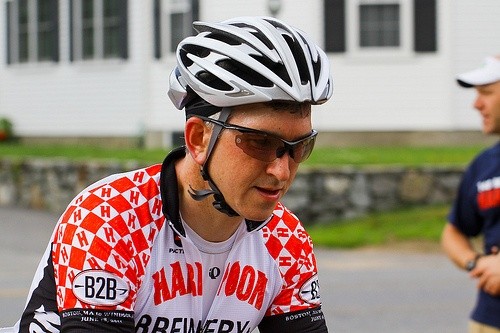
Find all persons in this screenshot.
[14,15,328,333]
[440,56,500,333]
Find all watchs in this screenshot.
[466,253,484,273]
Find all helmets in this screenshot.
[168,17,333,108]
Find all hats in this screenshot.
[457,56,500,86]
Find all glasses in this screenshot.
[186,114,317,162]
